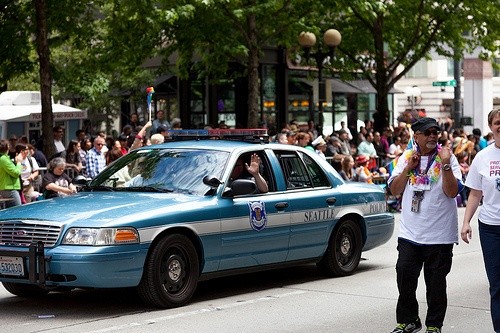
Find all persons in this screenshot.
[8,110,495,213]
[230,152,269,193]
[461,108,500,333]
[0,138,24,207]
[42,157,77,200]
[388,117,465,333]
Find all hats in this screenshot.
[286,131,296,136]
[289,125,300,132]
[356,155,367,163]
[411,117,441,132]
[311,135,326,147]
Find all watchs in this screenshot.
[441,163,451,171]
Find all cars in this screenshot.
[0,140,397,311]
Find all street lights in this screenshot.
[298,28,342,135]
[404,85,422,125]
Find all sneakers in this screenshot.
[390,316,422,333]
[425,326,441,333]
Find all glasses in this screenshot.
[56,130,64,133]
[417,130,437,136]
[96,143,105,146]
